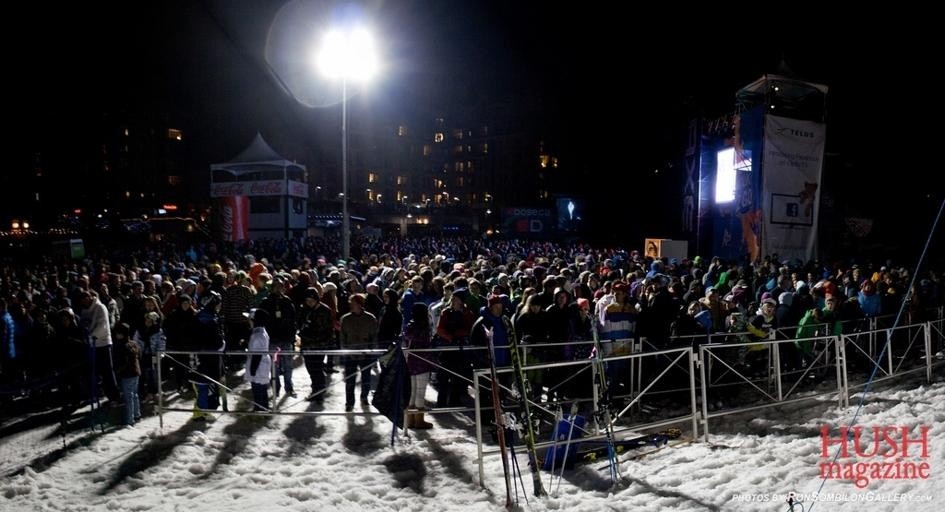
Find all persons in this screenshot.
[2,235,944,427]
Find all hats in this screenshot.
[242,307,260,319]
[797,280,805,291]
[322,283,338,293]
[513,270,533,290]
[534,267,546,279]
[179,294,192,305]
[723,295,738,304]
[453,286,469,300]
[152,274,162,285]
[435,254,446,261]
[272,278,284,288]
[577,297,590,308]
[760,292,799,306]
[305,287,319,303]
[366,284,379,293]
[299,272,313,284]
[612,283,627,293]
[589,273,600,282]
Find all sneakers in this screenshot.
[194,414,215,422]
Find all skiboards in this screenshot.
[503,314,681,496]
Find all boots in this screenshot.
[408,405,433,429]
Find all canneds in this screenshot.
[213,195,250,242]
